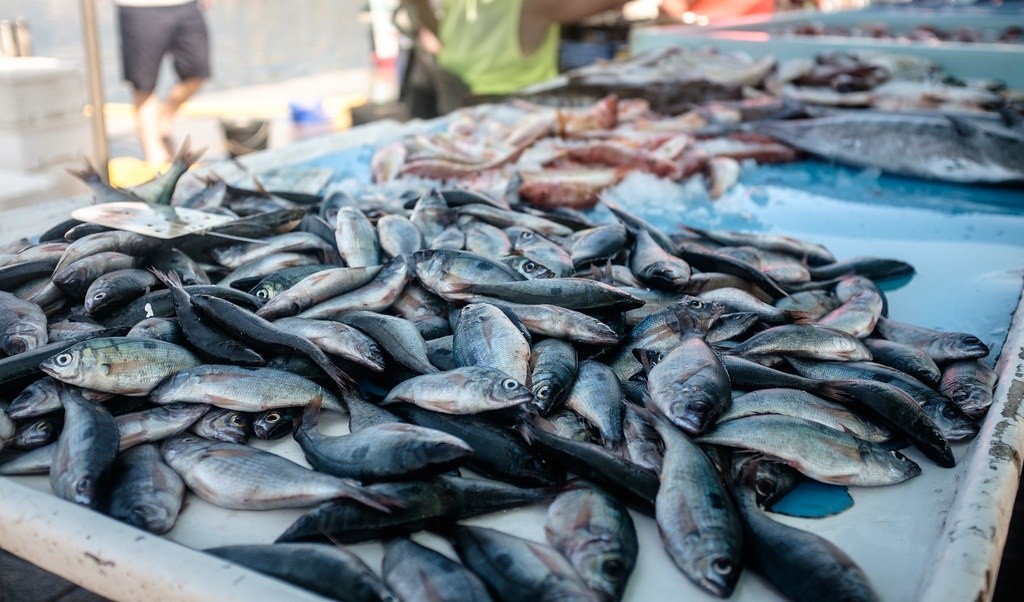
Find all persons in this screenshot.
[402,0,628,113]
[116,0,210,161]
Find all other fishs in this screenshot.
[565,47,1024,182]
[369,93,796,207]
[0,134,1024,602]
